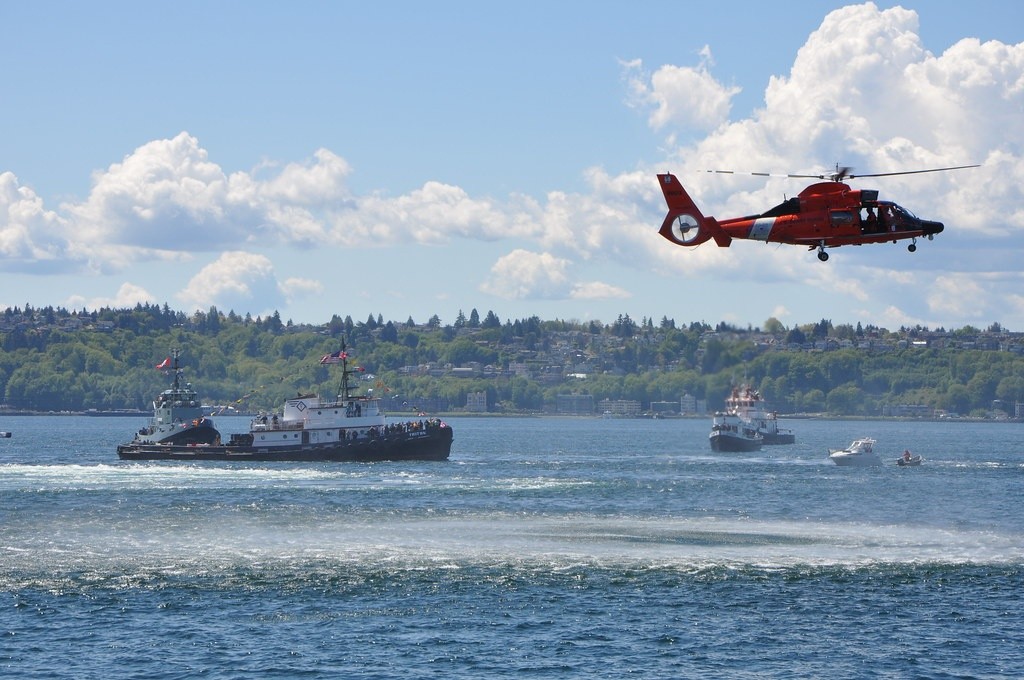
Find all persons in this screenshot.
[260,414,267,424]
[903,449,911,463]
[866,207,877,232]
[345,417,441,440]
[271,414,278,424]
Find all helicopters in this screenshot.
[655,161,980,262]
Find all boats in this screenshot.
[707,359,796,453]
[827,436,882,467]
[896,455,923,467]
[115,334,454,461]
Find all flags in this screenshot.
[320,349,341,364]
[156,357,170,369]
